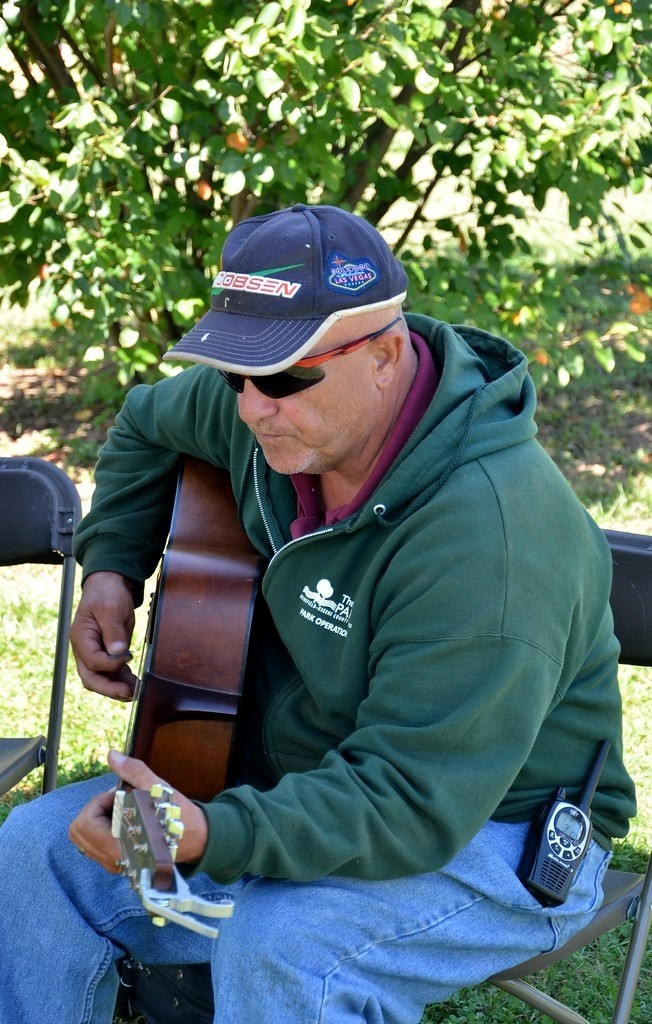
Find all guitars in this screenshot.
[112,454,266,939]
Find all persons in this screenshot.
[1,204,638,1024]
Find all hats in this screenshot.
[163,204,408,375]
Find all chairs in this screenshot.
[472,516,649,1024]
[0,454,82,826]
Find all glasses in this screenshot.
[218,318,403,398]
